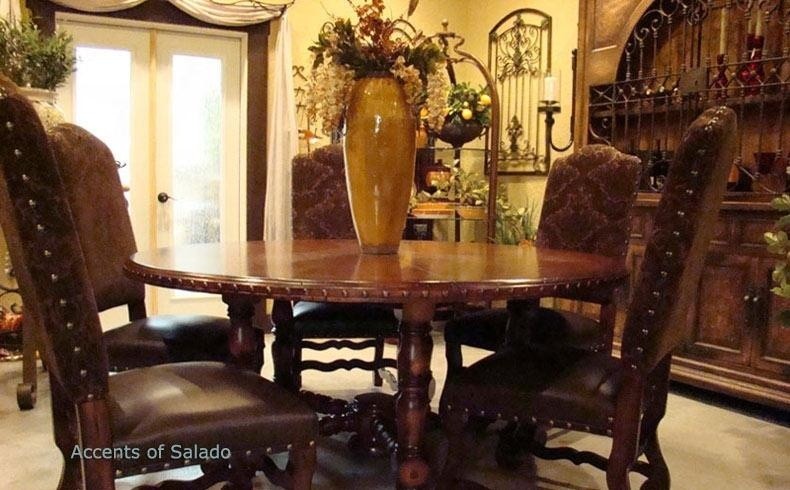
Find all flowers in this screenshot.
[298,0,450,129]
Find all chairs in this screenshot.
[45,121,228,364]
[0,72,360,490]
[439,107,737,489]
[279,141,428,399]
[445,144,643,398]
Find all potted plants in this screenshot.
[0,11,83,131]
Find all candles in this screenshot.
[748,20,752,34]
[544,71,558,102]
[755,12,761,38]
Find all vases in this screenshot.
[342,71,418,257]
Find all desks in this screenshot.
[120,233,635,489]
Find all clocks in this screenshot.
[716,8,728,56]
